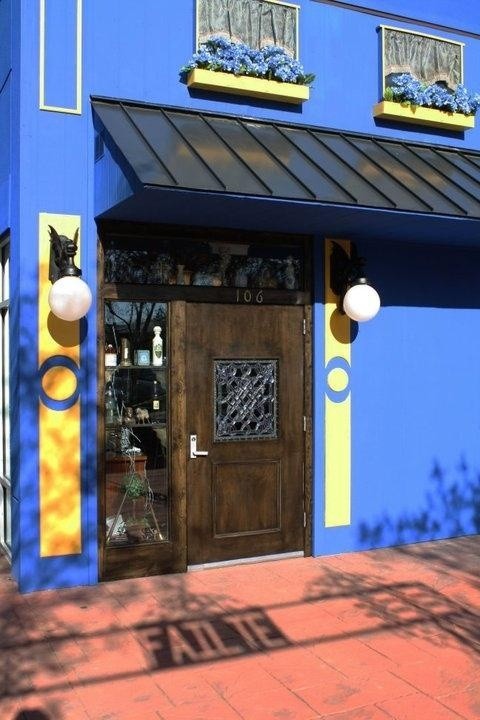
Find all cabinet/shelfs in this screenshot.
[105,300,169,550]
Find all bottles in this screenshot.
[151,326,164,367]
[151,380,162,424]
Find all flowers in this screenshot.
[178,34,314,84]
[384,71,480,116]
